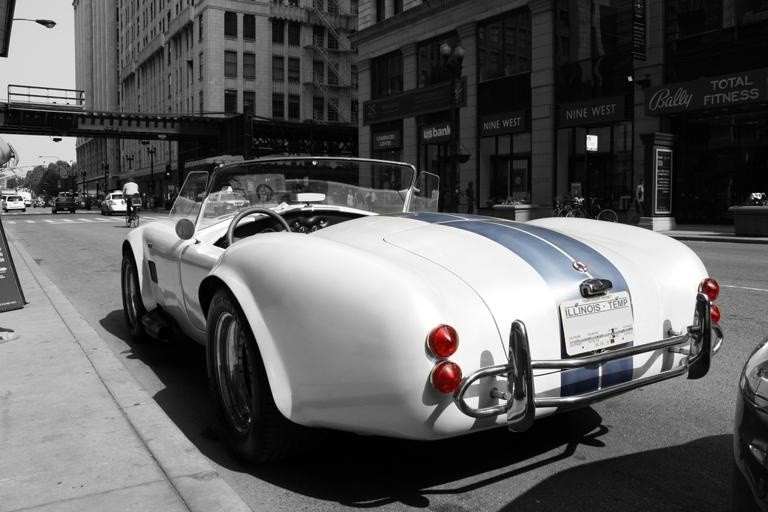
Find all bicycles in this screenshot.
[124,192,143,228]
[550,194,620,223]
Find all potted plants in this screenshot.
[490,194,539,224]
[725,195,767,237]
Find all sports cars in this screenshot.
[119,149,724,450]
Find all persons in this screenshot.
[451,183,464,212]
[465,180,477,214]
[633,178,644,215]
[122,177,139,223]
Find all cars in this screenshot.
[52,195,78,215]
[2,194,26,213]
[100,192,133,216]
